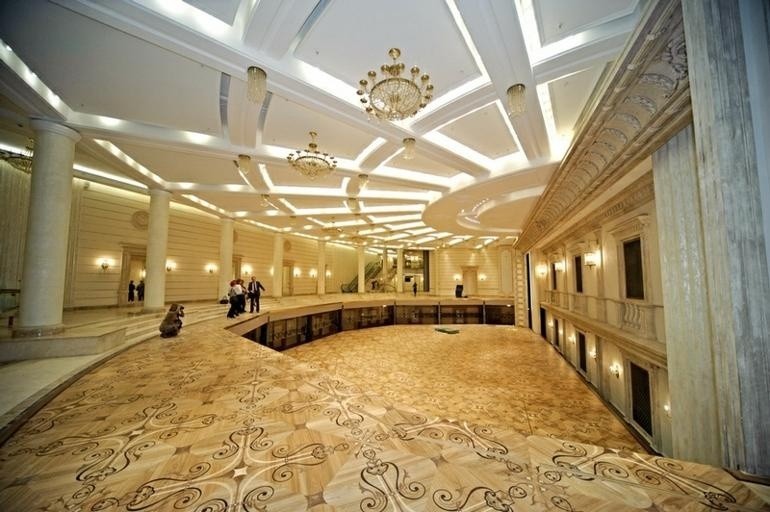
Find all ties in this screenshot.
[253,284,257,293]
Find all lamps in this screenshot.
[555,254,621,380]
[285,129,337,180]
[353,47,435,124]
[100,262,110,272]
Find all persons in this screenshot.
[136,280,144,300]
[129,280,135,305]
[248,276,265,313]
[413,282,417,297]
[159,303,184,338]
[227,278,247,318]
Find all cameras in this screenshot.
[180,305,184,310]
